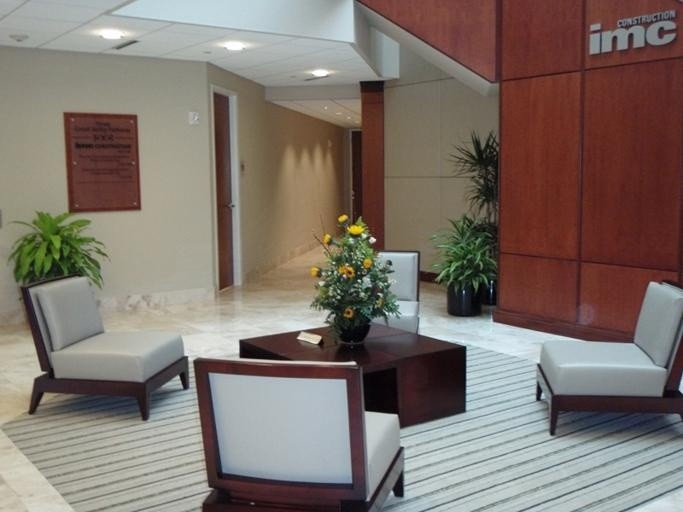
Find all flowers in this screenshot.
[310,213,403,342]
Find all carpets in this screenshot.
[2,343,682,512]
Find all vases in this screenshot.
[334,315,371,348]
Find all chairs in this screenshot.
[535,281,682,436]
[19,272,189,421]
[192,358,404,511]
[362,249,420,335]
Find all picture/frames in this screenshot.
[64,110,142,213]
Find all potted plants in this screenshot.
[426,127,500,318]
